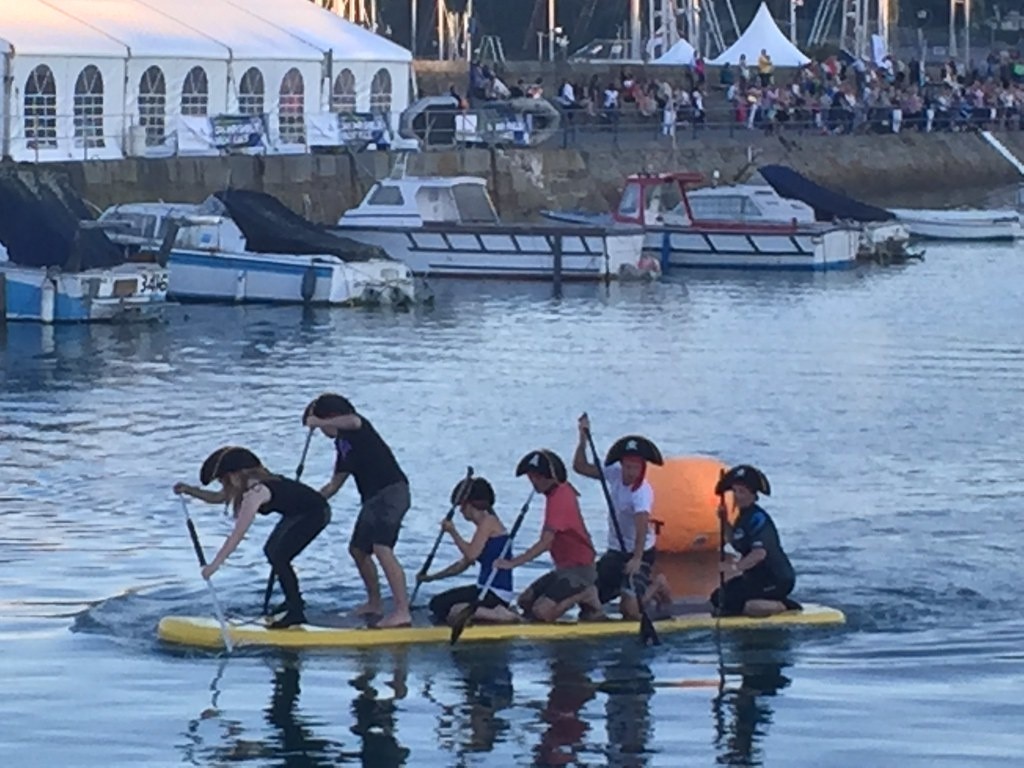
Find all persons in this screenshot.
[174,445,332,630]
[493,448,603,622]
[467,47,1024,137]
[709,465,804,619]
[370,119,389,151]
[573,415,671,621]
[303,393,413,628]
[450,84,461,108]
[415,478,530,626]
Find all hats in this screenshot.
[302,394,355,427]
[451,478,495,506]
[604,435,663,468]
[715,465,770,495]
[200,447,260,486]
[515,449,567,484]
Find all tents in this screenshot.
[702,0,812,66]
[646,38,701,65]
[0,0,419,152]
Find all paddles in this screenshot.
[174,482,236,657]
[259,423,318,616]
[449,484,536,648]
[408,463,474,612]
[713,467,727,636]
[577,412,662,647]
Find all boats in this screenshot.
[540,171,862,271]
[0,153,180,322]
[744,164,912,268]
[335,173,646,281]
[100,190,417,308]
[886,202,1024,241]
[398,95,563,150]
